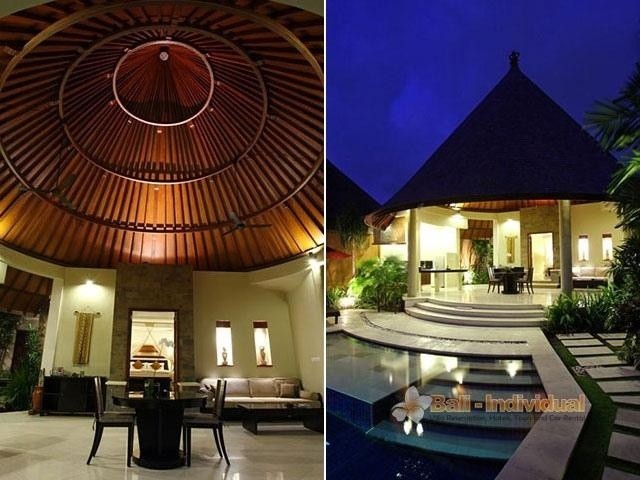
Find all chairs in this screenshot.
[487,264,534,294]
[87,376,230,467]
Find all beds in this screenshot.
[238,401,321,435]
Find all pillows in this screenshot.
[200,384,216,399]
[279,383,298,398]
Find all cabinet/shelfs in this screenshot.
[43,376,107,416]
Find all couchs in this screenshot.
[201,377,319,413]
[559,267,611,287]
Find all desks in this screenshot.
[420,267,468,292]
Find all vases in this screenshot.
[32,386,44,414]
